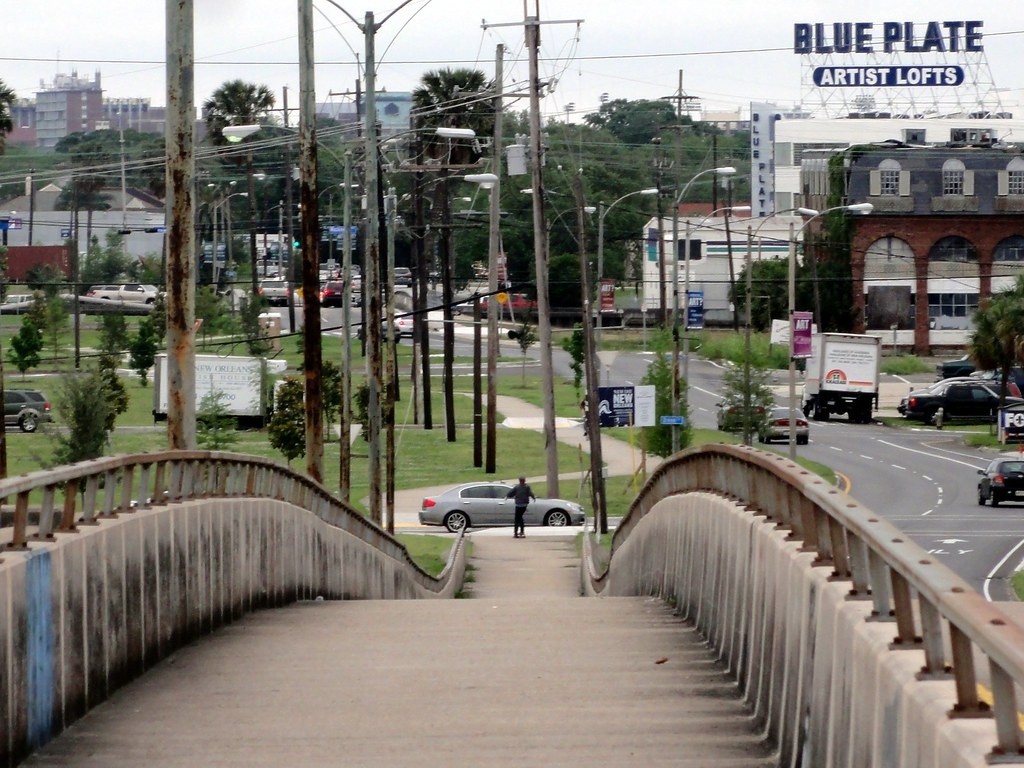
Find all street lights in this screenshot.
[218,120,478,512]
[784,201,875,461]
[544,204,598,323]
[669,164,737,453]
[210,191,251,290]
[742,206,819,447]
[595,188,663,406]
[682,204,751,405]
[385,171,500,535]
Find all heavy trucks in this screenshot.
[798,332,881,424]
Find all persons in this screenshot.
[505,475,538,538]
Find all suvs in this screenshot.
[898,377,994,421]
[910,381,1016,428]
[1,388,54,434]
[86,285,120,302]
[117,285,168,307]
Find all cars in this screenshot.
[201,237,540,345]
[976,456,1024,509]
[418,483,585,534]
[936,353,1024,398]
[756,407,809,445]
[714,393,764,431]
[2,294,36,312]
